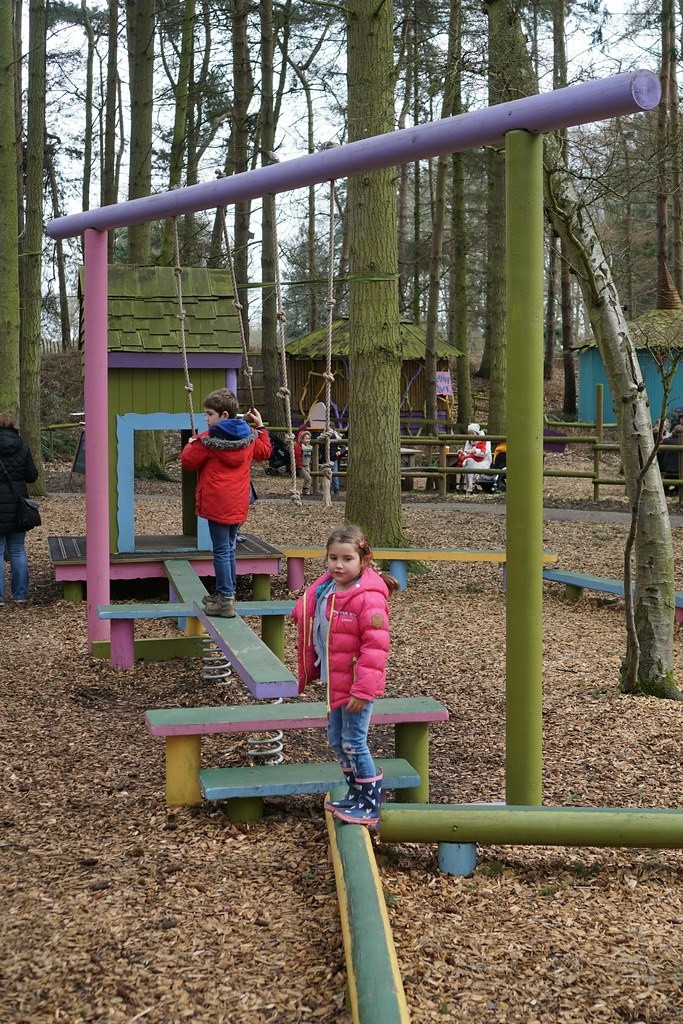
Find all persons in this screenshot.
[290,525,401,825]
[294,431,313,497]
[649,414,683,501]
[318,421,347,493]
[462,423,493,495]
[0,413,42,606]
[458,431,486,469]
[180,388,272,617]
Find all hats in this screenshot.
[468,424,480,432]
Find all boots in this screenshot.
[334,767,383,828]
[202,591,236,605]
[324,768,362,812]
[205,593,236,618]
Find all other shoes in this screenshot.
[14,599,28,603]
[298,431,311,442]
[303,488,310,494]
[0,602,4,605]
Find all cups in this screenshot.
[446,446,450,453]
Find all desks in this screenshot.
[401,447,421,490]
[425,451,464,494]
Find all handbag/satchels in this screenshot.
[17,497,41,529]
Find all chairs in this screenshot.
[476,442,507,494]
